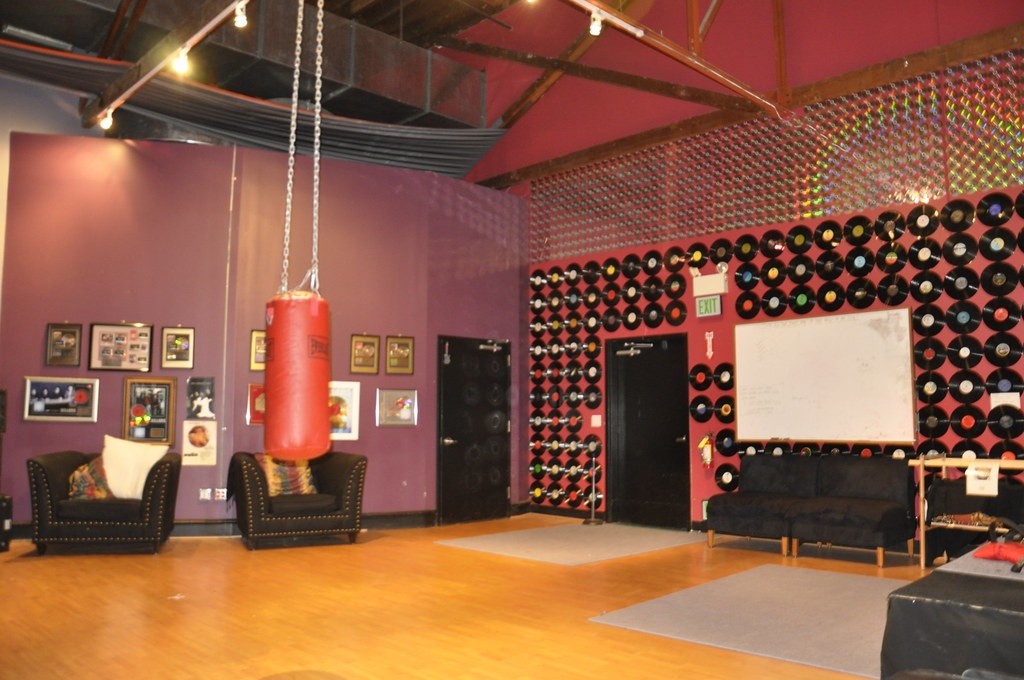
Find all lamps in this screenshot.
[234,4,248,28]
[688,261,730,297]
[100,109,115,130]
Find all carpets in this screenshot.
[435,521,720,566]
[591,563,913,678]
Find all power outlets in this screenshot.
[215,489,227,500]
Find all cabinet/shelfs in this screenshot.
[908,455,1024,570]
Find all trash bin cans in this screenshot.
[0,495,13,553]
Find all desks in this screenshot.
[881,540,1024,680]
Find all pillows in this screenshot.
[103,435,169,500]
[254,453,316,495]
[68,455,109,499]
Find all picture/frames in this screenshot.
[385,336,414,375]
[376,386,417,429]
[23,376,99,423]
[121,377,177,447]
[88,323,155,373]
[350,333,380,375]
[46,323,82,367]
[250,330,267,371]
[330,381,360,441]
[161,327,195,369]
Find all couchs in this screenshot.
[227,452,367,551]
[28,451,181,554]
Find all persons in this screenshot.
[187,389,216,419]
[29,384,76,413]
[137,388,162,416]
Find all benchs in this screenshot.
[705,454,916,568]
